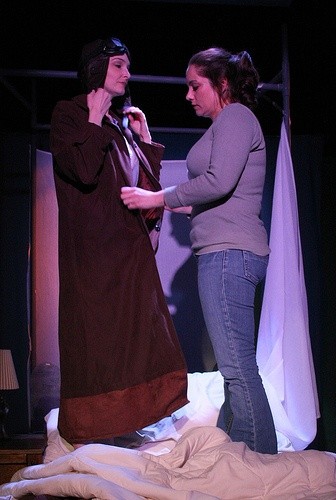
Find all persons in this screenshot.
[121,46,279,457]
[49,37,189,463]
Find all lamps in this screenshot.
[0,350,20,438]
